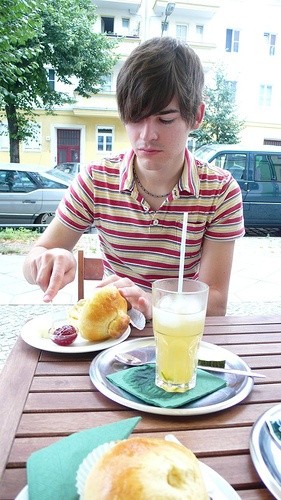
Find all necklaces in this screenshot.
[132,161,171,198]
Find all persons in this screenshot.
[23,36,245,316]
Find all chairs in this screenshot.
[229,156,246,178]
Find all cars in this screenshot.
[0,163,93,234]
[53,162,87,176]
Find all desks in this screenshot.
[0,313,281,500]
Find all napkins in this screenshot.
[26,415,143,500]
[106,362,227,409]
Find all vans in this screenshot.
[192,145,281,230]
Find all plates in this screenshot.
[89,337,255,416]
[14,461,240,500]
[21,312,131,353]
[249,404,281,500]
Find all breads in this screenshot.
[82,436,211,500]
[71,284,131,341]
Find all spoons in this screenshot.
[116,353,267,378]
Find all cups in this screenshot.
[152,277,209,392]
[49,324,79,344]
